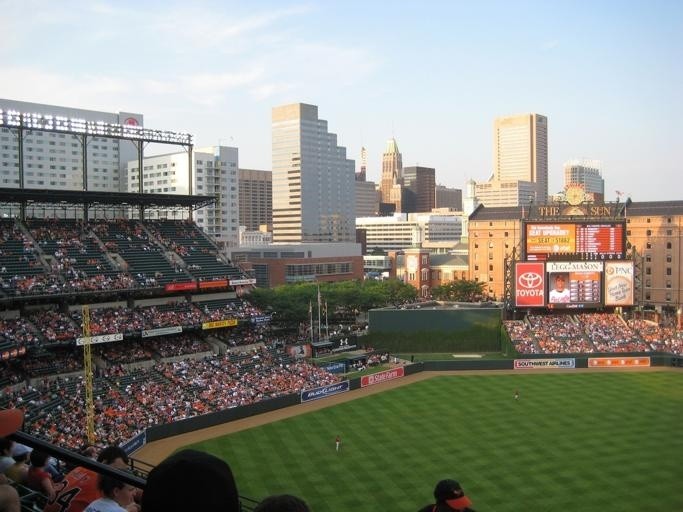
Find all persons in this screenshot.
[513,387,519,403]
[502,313,683,356]
[430,480,476,511]
[549,274,570,305]
[0,345,344,451]
[0,298,269,373]
[0,452,310,512]
[352,351,388,372]
[0,216,245,295]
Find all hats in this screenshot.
[434,478,473,510]
